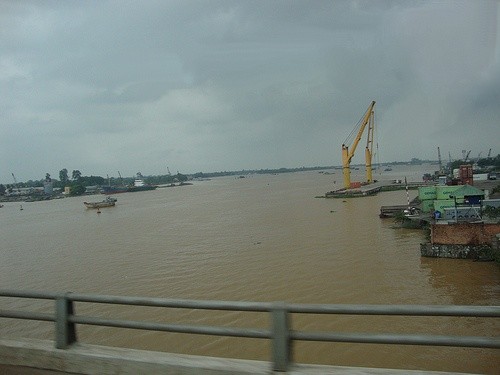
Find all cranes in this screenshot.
[339,102,376,190]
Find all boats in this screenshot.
[83,197,117,207]
[317,165,392,175]
[98,177,156,194]
